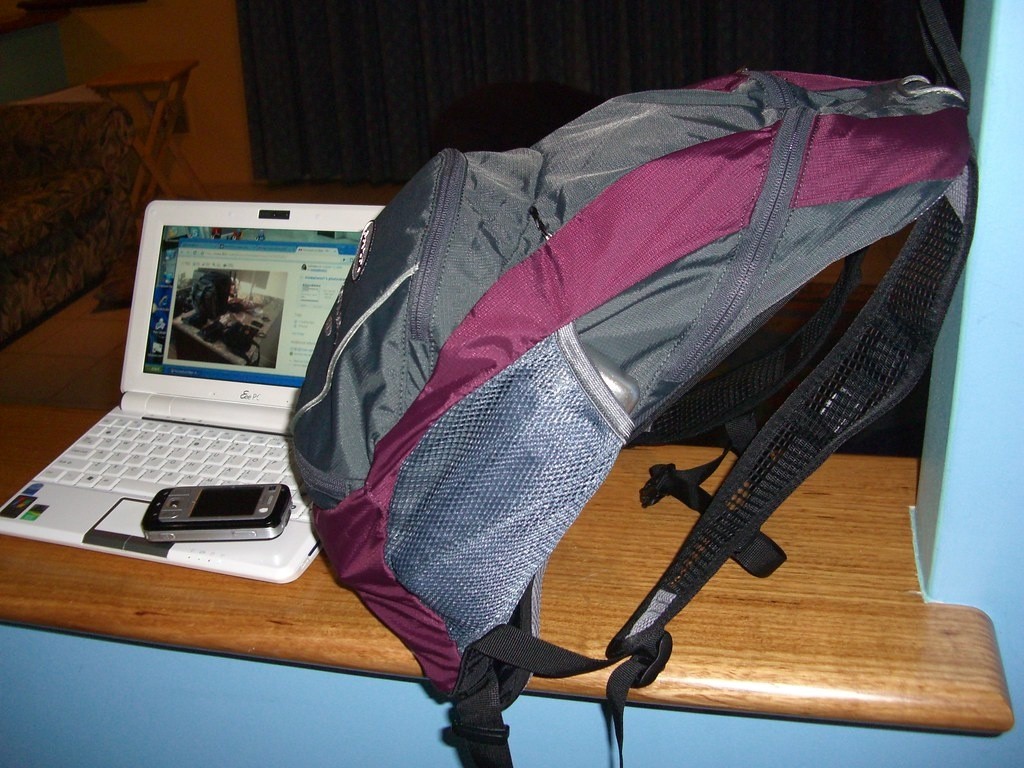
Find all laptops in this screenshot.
[0,200,384,584]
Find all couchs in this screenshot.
[0,103,140,343]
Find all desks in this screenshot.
[0,400,1013,738]
[85,59,210,243]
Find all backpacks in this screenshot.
[288,67,977,767]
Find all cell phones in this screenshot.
[141,483,291,542]
